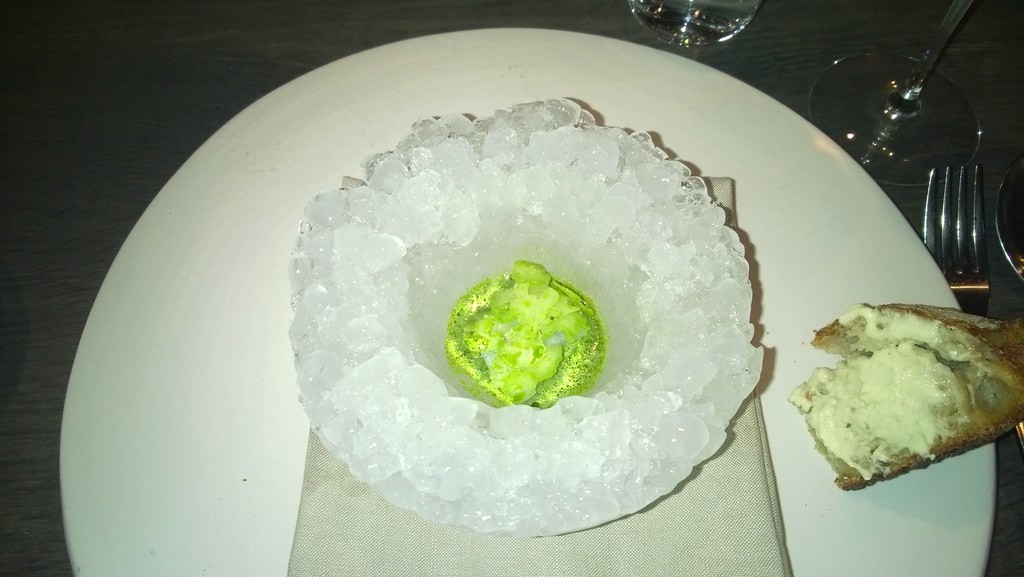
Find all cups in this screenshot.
[627,0,763,46]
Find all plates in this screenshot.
[59,27,998,577]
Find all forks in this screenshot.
[920,163,1024,456]
